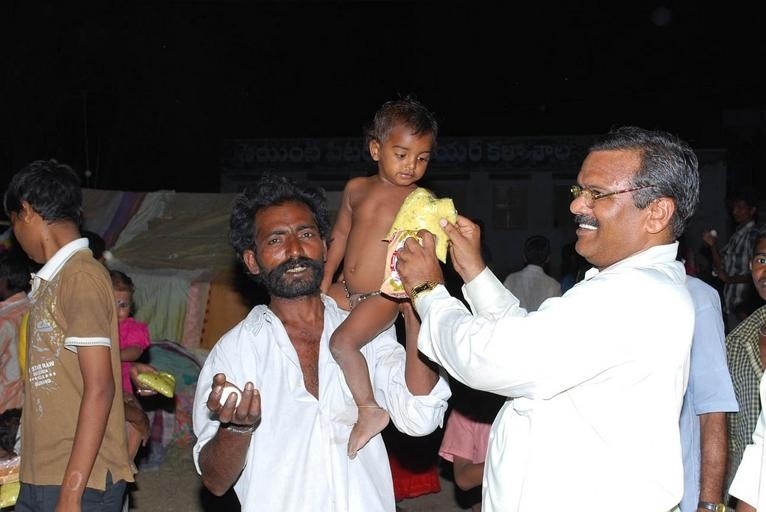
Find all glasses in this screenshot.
[569,184,657,209]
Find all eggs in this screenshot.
[220,387,242,410]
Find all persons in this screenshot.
[395,123,700,511]
[191,167,453,512]
[1,156,140,512]
[320,95,439,461]
[0,190,764,512]
